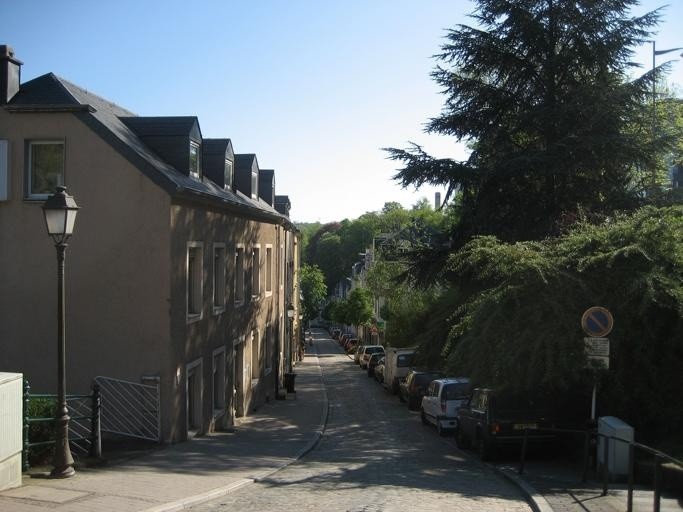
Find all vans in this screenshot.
[419,376,476,437]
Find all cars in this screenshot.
[398,370,443,410]
[325,325,384,384]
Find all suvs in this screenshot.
[456,387,562,463]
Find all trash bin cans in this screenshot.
[285,373,298,393]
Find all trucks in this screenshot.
[383,346,420,395]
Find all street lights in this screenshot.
[298,312,303,336]
[651,41,682,186]
[286,302,295,375]
[39,183,83,481]
